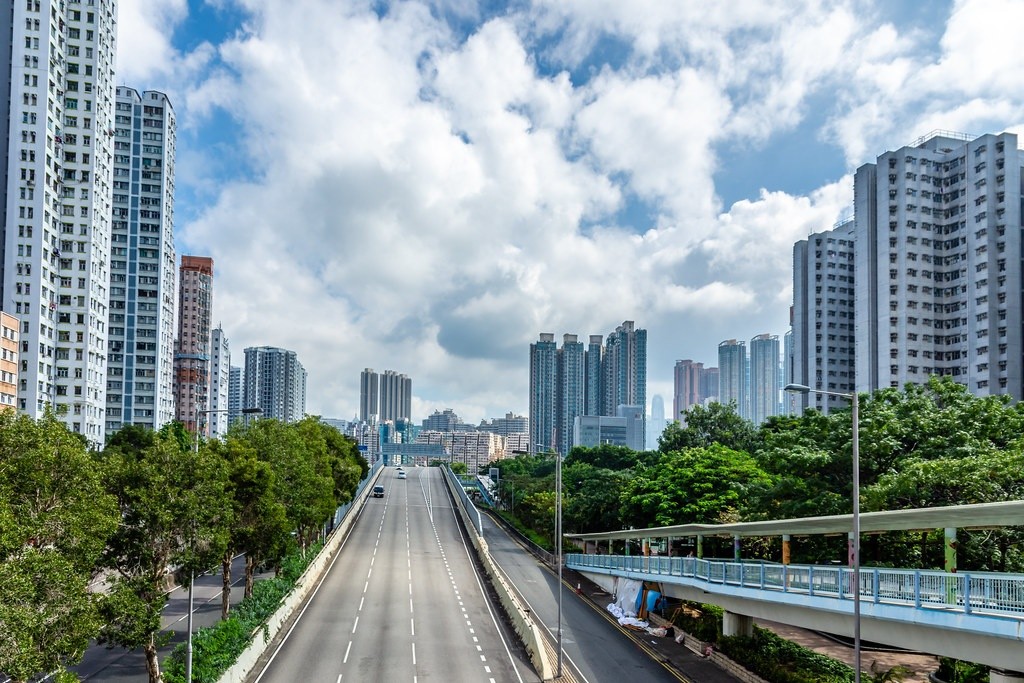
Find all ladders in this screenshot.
[665,608,681,628]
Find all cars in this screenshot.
[396,465,403,470]
[373,485,385,498]
[397,471,408,479]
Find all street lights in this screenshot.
[511,450,564,678]
[784,383,861,683]
[182,407,265,683]
